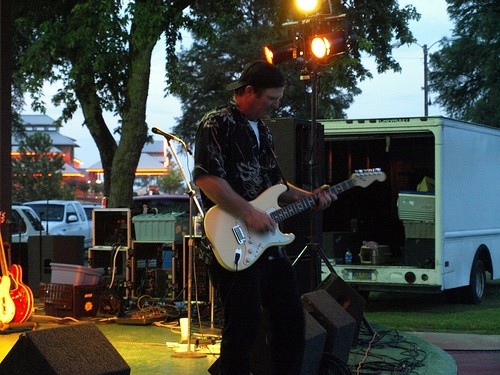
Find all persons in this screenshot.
[193,60,338,375]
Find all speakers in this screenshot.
[28,235,84,297]
[260,115,365,373]
[0,321,131,375]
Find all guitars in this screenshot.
[0,211,34,324]
[202,168,387,272]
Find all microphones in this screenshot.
[151,128,182,142]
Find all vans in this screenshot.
[20,200,102,251]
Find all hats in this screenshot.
[226,60,285,91]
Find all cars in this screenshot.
[9,204,51,267]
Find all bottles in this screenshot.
[102,196,106,208]
[194,216,202,237]
[345,250,352,265]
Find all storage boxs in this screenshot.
[396,192,435,223]
[47,263,101,286]
[358,246,390,264]
[132,213,186,240]
[42,283,104,318]
[402,221,435,266]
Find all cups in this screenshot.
[180,317,188,341]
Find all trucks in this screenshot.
[309,115,500,307]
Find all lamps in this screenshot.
[263,0,358,66]
